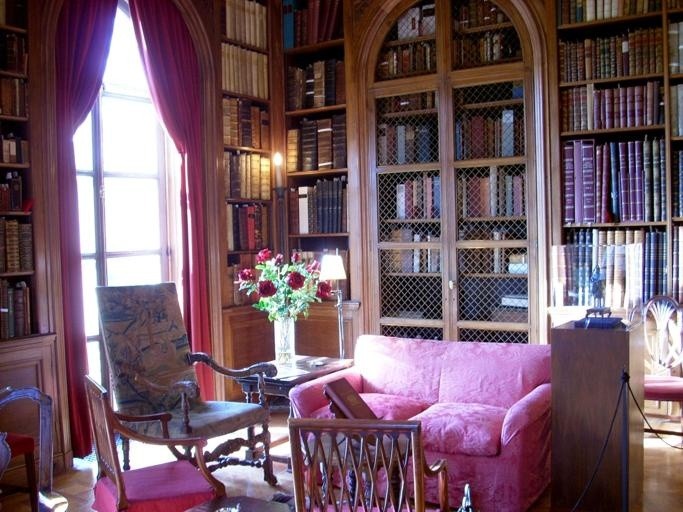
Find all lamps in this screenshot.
[270,152,291,203]
[315,252,348,358]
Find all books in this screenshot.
[283,0,351,298]
[0,0,38,342]
[556,0,682,309]
[374,3,528,276]
[324,376,380,426]
[221,0,258,222]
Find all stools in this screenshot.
[0,428,38,512]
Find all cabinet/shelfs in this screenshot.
[0,0,67,360]
[546,310,647,512]
[367,0,540,344]
[277,0,359,311]
[213,0,289,314]
[551,0,683,337]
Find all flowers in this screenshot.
[233,245,332,320]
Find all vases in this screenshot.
[268,316,298,370]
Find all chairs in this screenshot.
[0,383,70,512]
[285,416,452,511]
[81,369,229,512]
[631,295,682,447]
[89,280,279,492]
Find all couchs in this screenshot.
[286,333,553,511]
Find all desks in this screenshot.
[234,355,357,475]
[299,429,417,512]
[175,495,292,512]
